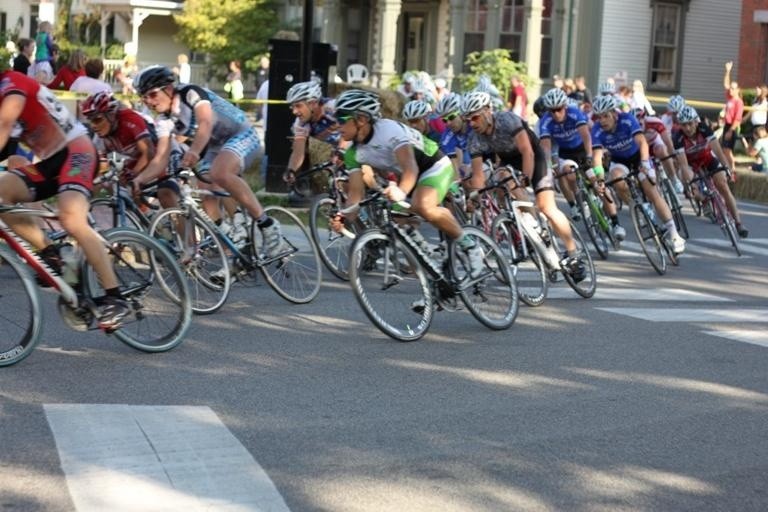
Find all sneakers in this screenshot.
[98,292,130,331]
[262,215,281,258]
[467,239,487,279]
[570,204,581,222]
[413,296,445,316]
[736,221,749,239]
[671,176,686,195]
[208,266,237,286]
[614,224,626,241]
[36,242,63,289]
[702,199,713,215]
[570,250,585,285]
[217,215,251,246]
[671,234,685,254]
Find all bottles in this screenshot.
[406,225,433,257]
[214,217,231,237]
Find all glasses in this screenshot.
[440,110,460,123]
[337,114,353,124]
[90,112,107,124]
[141,85,167,101]
[464,114,481,122]
[289,101,309,109]
[551,104,566,113]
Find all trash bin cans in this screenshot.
[265,36,334,193]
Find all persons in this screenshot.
[0,21,767,328]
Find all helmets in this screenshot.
[132,64,179,96]
[435,92,461,116]
[403,71,446,93]
[533,88,577,113]
[333,89,381,117]
[80,91,117,117]
[667,94,685,113]
[472,83,500,97]
[460,93,488,116]
[592,96,616,114]
[401,99,432,121]
[628,108,644,121]
[678,106,698,123]
[286,80,321,106]
[600,81,616,94]
